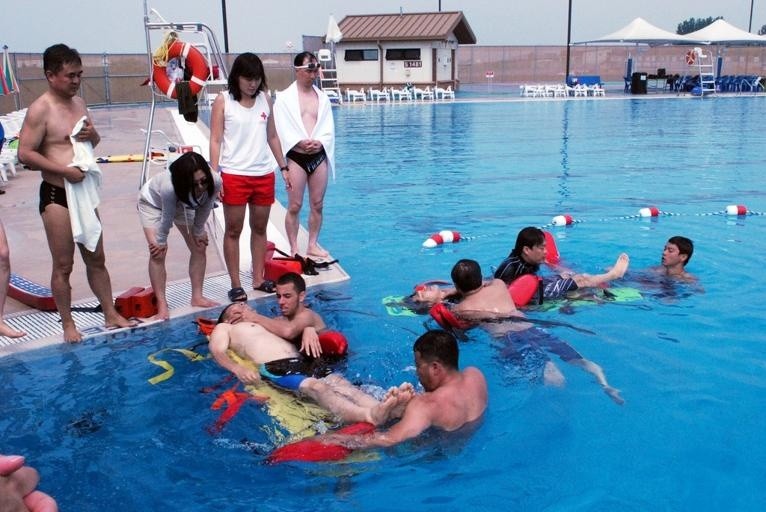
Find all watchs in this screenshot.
[280,165,290,171]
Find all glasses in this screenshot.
[193,178,207,187]
[294,63,320,70]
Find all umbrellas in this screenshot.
[572,18,711,73]
[683,18,766,76]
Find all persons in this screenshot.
[209,52,296,303]
[399,224,630,314]
[313,467,370,498]
[0,123,30,340]
[17,44,139,346]
[209,301,415,427]
[0,453,60,512]
[380,328,488,440]
[603,235,704,307]
[229,272,326,340]
[271,51,338,264]
[135,150,223,320]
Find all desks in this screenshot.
[647,76,672,93]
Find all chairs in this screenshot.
[345,87,454,101]
[672,73,765,92]
[520,83,605,97]
[1,108,27,182]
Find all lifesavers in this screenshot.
[153,42,207,97]
[686,49,696,64]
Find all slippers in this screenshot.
[253,280,275,292]
[227,288,246,302]
